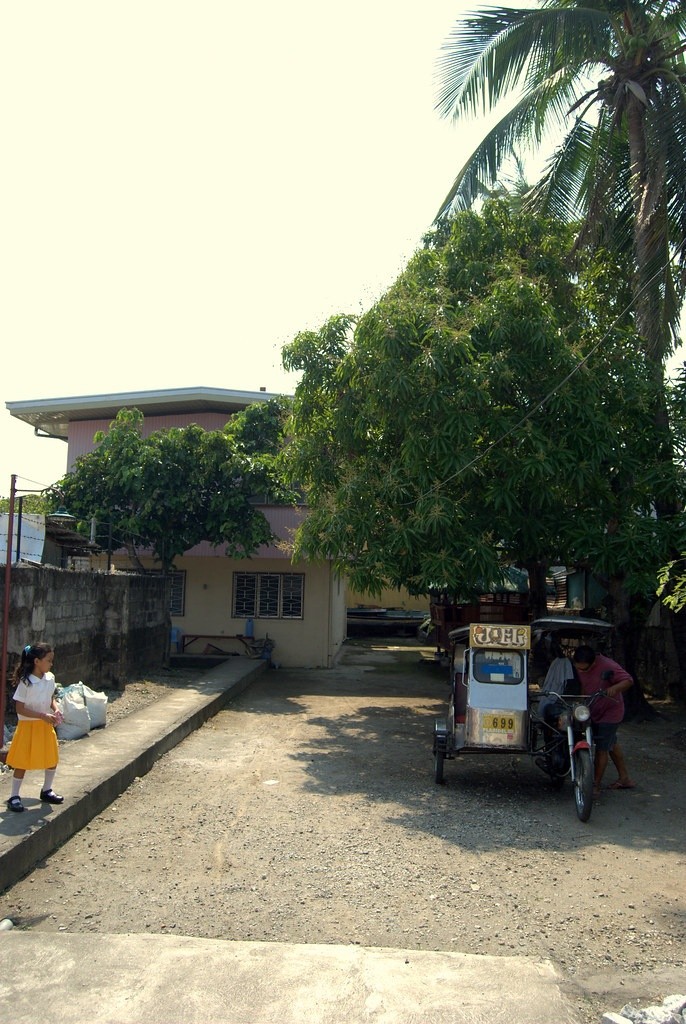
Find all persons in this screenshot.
[573,646,637,796]
[5,643,63,813]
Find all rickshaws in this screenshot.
[433,615,622,824]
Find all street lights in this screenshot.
[0,475,79,745]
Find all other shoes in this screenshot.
[608,777,636,790]
[7,796,25,812]
[592,778,602,800]
[40,790,64,803]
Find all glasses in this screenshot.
[576,664,592,671]
